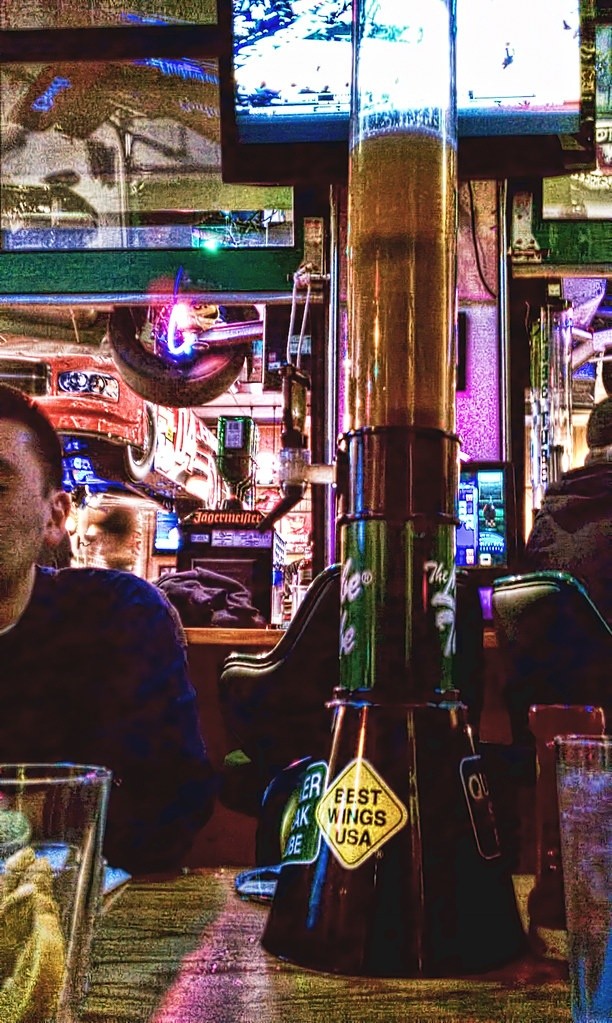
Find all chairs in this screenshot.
[488,572,611,761]
[220,564,483,817]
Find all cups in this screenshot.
[0,761,112,1023]
[553,735,612,1023]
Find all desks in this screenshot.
[69,865,576,1022]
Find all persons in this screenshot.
[483,502,497,528]
[524,398,612,619]
[0,382,214,872]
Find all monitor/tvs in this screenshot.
[216,0,596,188]
[458,461,524,578]
